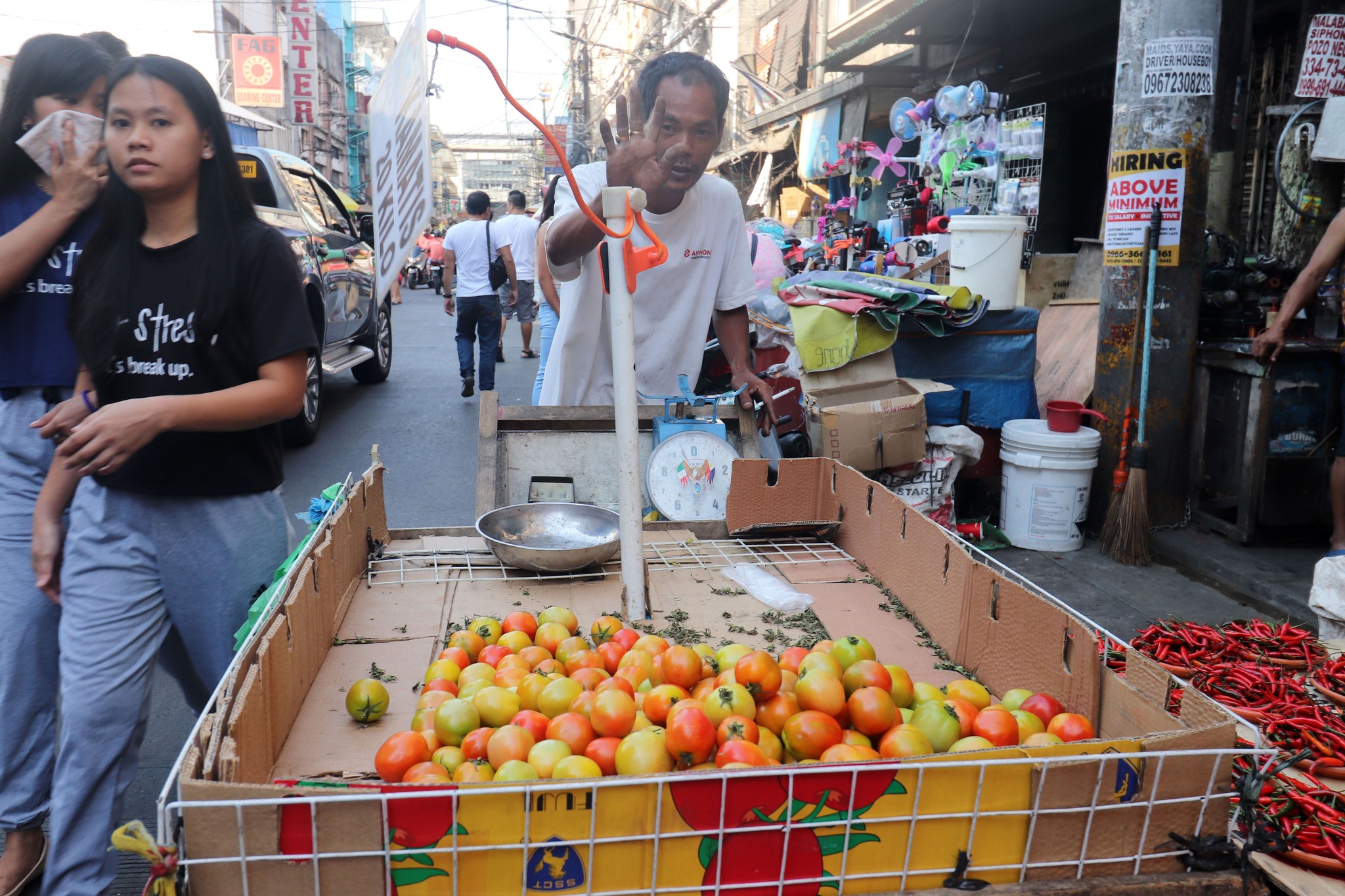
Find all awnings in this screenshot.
[802,0,985,73]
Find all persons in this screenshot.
[1,32,121,896]
[539,51,778,438]
[494,190,540,362]
[1252,209,1345,558]
[400,224,446,287]
[532,173,563,406]
[442,190,518,397]
[34,53,318,895]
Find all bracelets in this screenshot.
[82,389,95,413]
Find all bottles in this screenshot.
[1265,298,1279,331]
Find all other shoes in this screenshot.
[461,372,474,397]
[427,273,432,282]
[2,833,49,896]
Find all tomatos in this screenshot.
[375,607,1094,785]
[346,678,388,721]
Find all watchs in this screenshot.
[443,292,452,298]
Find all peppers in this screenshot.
[1096,614,1345,863]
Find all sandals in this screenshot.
[520,350,540,358]
[496,340,505,362]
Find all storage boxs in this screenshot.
[177,185,1235,896]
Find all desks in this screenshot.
[1186,334,1345,547]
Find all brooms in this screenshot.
[1096,208,1168,566]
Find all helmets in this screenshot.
[433,230,441,237]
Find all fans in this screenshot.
[864,80,1009,198]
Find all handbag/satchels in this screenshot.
[488,255,508,291]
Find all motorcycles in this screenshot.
[429,257,445,295]
[406,245,431,290]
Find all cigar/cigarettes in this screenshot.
[757,386,795,406]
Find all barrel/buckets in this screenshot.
[999,418,1102,553]
[947,215,1027,311]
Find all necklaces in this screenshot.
[470,218,481,220]
[391,276,402,304]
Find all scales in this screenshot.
[637,374,747,520]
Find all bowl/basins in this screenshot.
[474,501,619,574]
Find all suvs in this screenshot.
[233,144,395,447]
